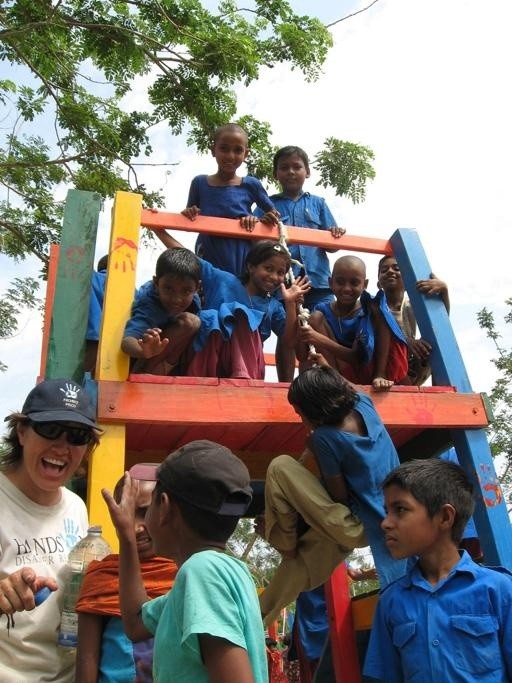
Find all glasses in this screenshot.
[24,418,95,447]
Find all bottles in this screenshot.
[59,525,112,650]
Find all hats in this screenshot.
[20,377,104,433]
[128,439,254,518]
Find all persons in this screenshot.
[258,354,510,682]
[0,378,269,683]
[87,125,449,388]
